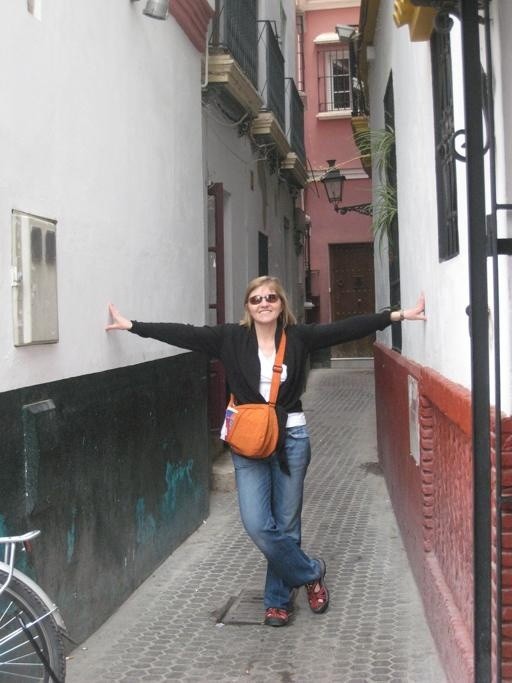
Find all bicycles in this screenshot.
[1,528,80,681]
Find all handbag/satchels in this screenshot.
[222,402,280,461]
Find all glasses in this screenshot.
[246,293,280,305]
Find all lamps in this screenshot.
[333,21,361,41]
[320,157,374,219]
[129,0,172,19]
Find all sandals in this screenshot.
[304,555,328,613]
[263,607,293,627]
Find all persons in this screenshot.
[105,276,424,626]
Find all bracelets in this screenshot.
[400,308,405,322]
